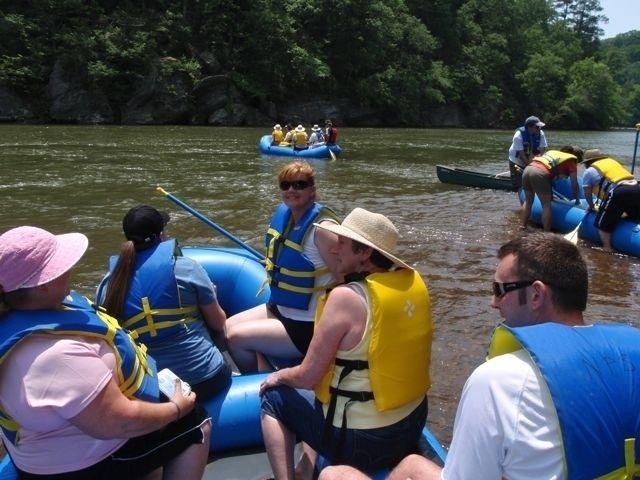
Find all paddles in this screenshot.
[563,199,598,246]
[320,132,336,160]
[279,141,292,146]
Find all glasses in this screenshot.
[493,280,534,298]
[280,180,312,191]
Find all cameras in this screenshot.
[158,368,191,400]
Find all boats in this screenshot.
[260,136,341,159]
[513,171,640,256]
[1,244,449,479]
[434,164,521,193]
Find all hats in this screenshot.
[312,207,414,271]
[572,145,584,163]
[0,225,88,294]
[525,115,546,127]
[580,149,608,163]
[123,204,171,244]
[273,123,321,132]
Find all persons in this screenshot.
[272,124,283,145]
[223,162,342,375]
[0,224,213,478]
[506,115,547,205]
[308,124,324,145]
[257,207,433,478]
[582,149,640,253]
[523,144,579,231]
[323,120,337,146]
[284,123,296,142]
[292,125,309,151]
[318,231,639,477]
[93,205,233,397]
